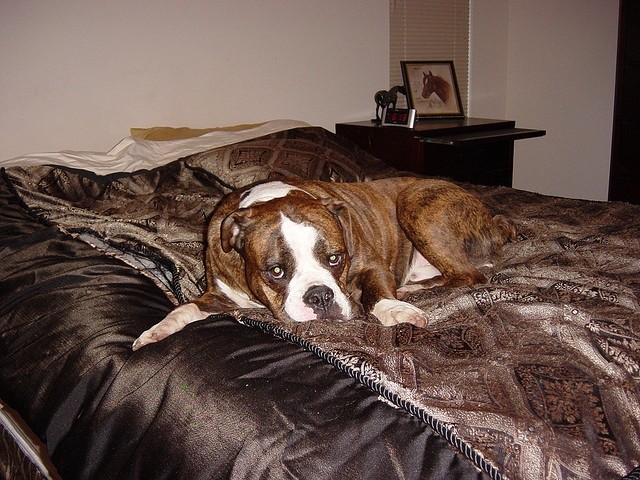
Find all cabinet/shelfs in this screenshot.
[336,117,546,188]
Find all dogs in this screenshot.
[131,175,519,353]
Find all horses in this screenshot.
[373,84,405,119]
[421,69,452,106]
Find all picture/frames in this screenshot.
[399,60,465,120]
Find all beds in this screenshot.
[0,117,640,480]
